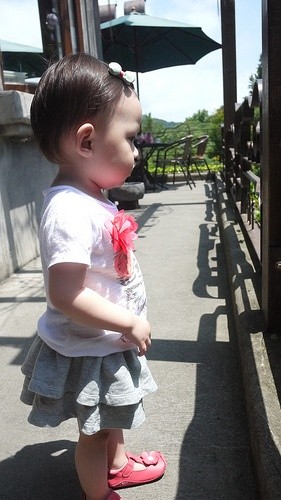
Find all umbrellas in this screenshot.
[0,40,43,73]
[100,7,222,133]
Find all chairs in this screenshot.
[153,135,213,192]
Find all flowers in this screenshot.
[104,209,139,278]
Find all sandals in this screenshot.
[108,451,165,487]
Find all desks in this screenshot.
[125,143,172,190]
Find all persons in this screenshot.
[19,54,168,500]
